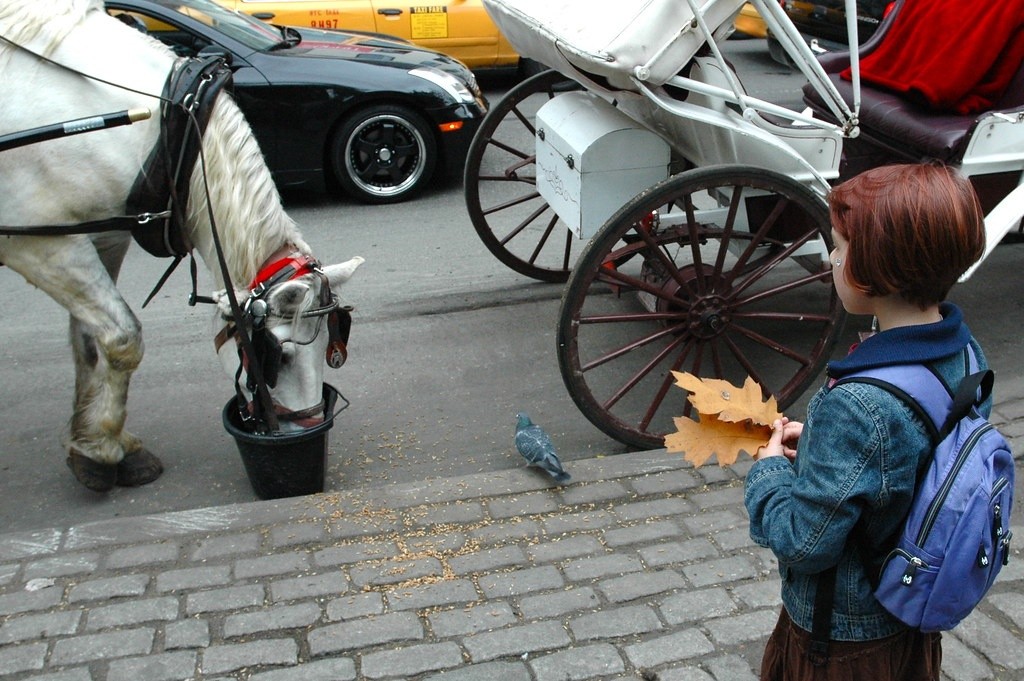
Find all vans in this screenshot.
[109,0,572,85]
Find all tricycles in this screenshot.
[464,0,1019,449]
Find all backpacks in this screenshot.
[833,341,1015,632]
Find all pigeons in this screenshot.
[515,413,571,482]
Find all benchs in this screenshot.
[481,0,842,181]
[803,0,1024,168]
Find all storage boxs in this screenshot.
[535,91,671,240]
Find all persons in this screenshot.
[744,161,1016,681]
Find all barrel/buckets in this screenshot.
[222,382,350,500]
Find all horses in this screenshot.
[0,0,364,491]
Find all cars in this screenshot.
[100,0,492,206]
[732,0,891,70]
